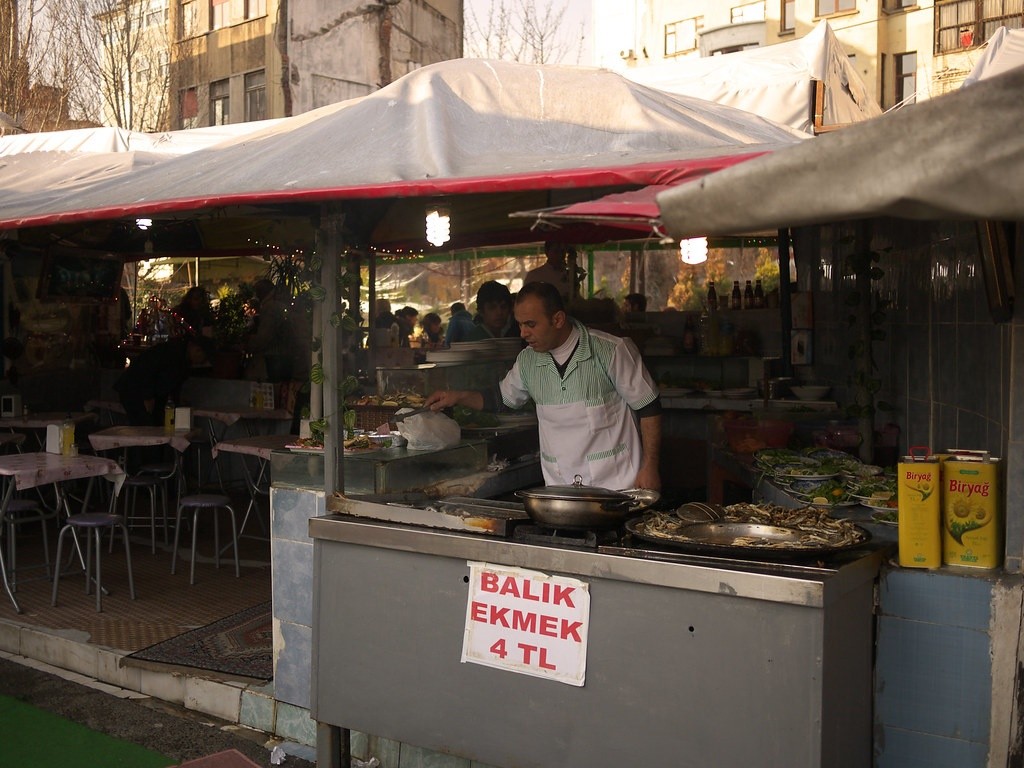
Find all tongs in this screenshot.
[388,403,436,424]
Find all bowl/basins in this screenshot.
[790,385,832,401]
[625,510,872,556]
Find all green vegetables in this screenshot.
[450,407,504,428]
[754,447,899,522]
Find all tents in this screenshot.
[0,58,813,490]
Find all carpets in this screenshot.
[125,600,272,683]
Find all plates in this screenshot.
[617,489,660,515]
[425,336,523,364]
[749,444,901,527]
[285,437,381,457]
[657,387,757,399]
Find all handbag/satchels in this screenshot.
[394,407,462,451]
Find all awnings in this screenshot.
[508,19,1024,245]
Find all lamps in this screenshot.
[679,236,709,265]
[425,195,450,247]
[136,219,153,230]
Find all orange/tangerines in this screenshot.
[813,497,828,503]
[872,492,890,499]
[790,470,803,475]
[760,455,769,460]
[832,490,844,496]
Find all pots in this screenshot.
[513,473,641,532]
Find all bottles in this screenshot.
[754,279,764,308]
[62,416,78,457]
[164,396,176,430]
[732,280,741,309]
[743,280,754,309]
[684,312,759,360]
[707,282,717,311]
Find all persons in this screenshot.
[112,335,217,494]
[423,283,663,492]
[119,239,649,364]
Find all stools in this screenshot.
[0,452,240,613]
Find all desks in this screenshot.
[84,397,155,426]
[91,425,202,543]
[208,434,303,559]
[0,452,129,597]
[190,407,290,497]
[100,369,283,412]
[1,410,100,508]
